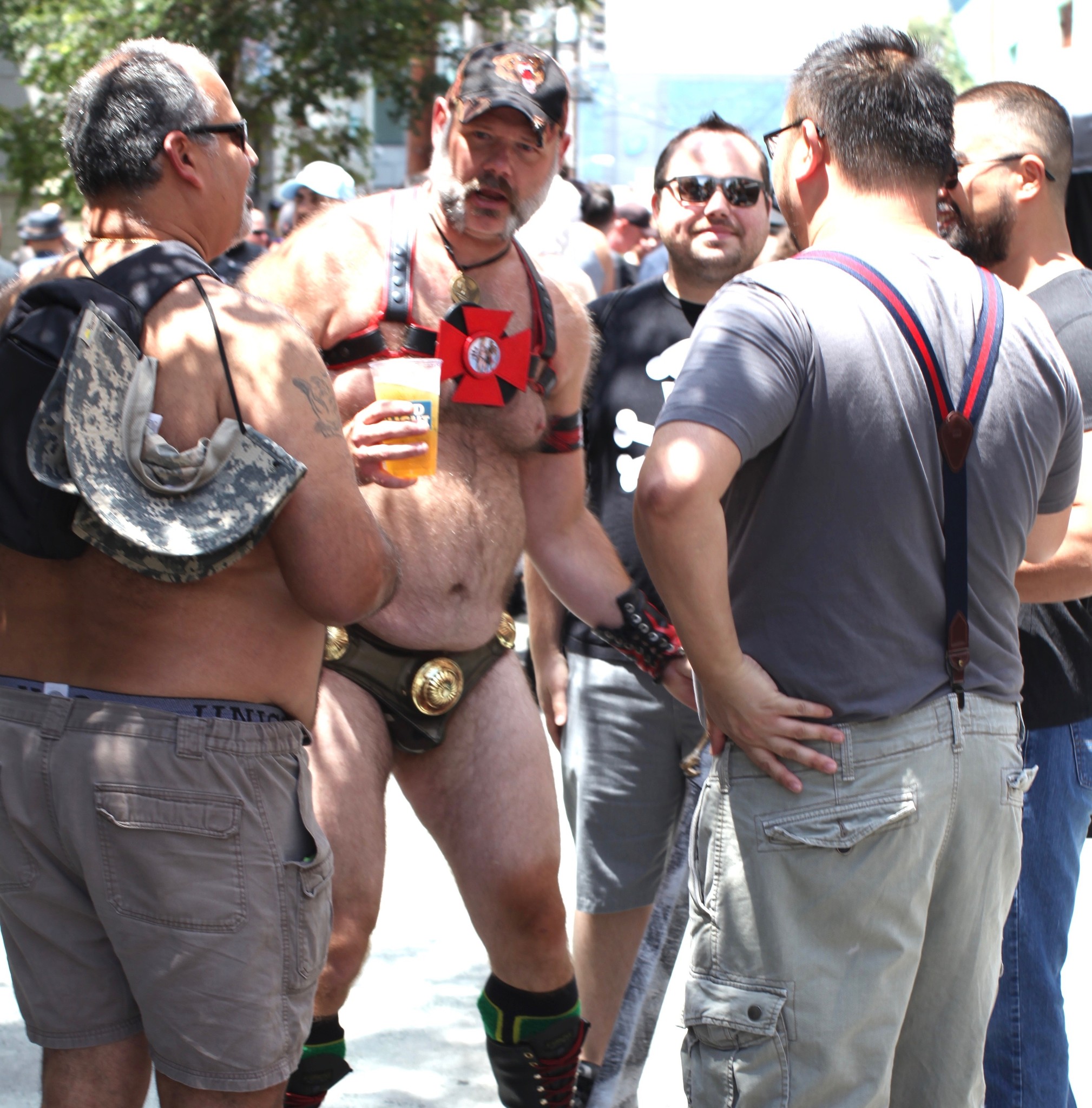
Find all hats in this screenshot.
[447,40,570,147]
[279,160,355,202]
[13,210,66,240]
[25,302,309,586]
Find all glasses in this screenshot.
[252,228,270,235]
[764,118,826,159]
[183,119,248,152]
[660,175,767,207]
[946,154,1056,190]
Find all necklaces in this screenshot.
[429,212,511,306]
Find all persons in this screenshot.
[630,25,1084,1108]
[1,33,401,1108]
[557,161,671,299]
[0,160,357,290]
[936,80,1092,1108]
[232,41,700,1108]
[521,112,802,1108]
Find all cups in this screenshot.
[367,358,443,478]
